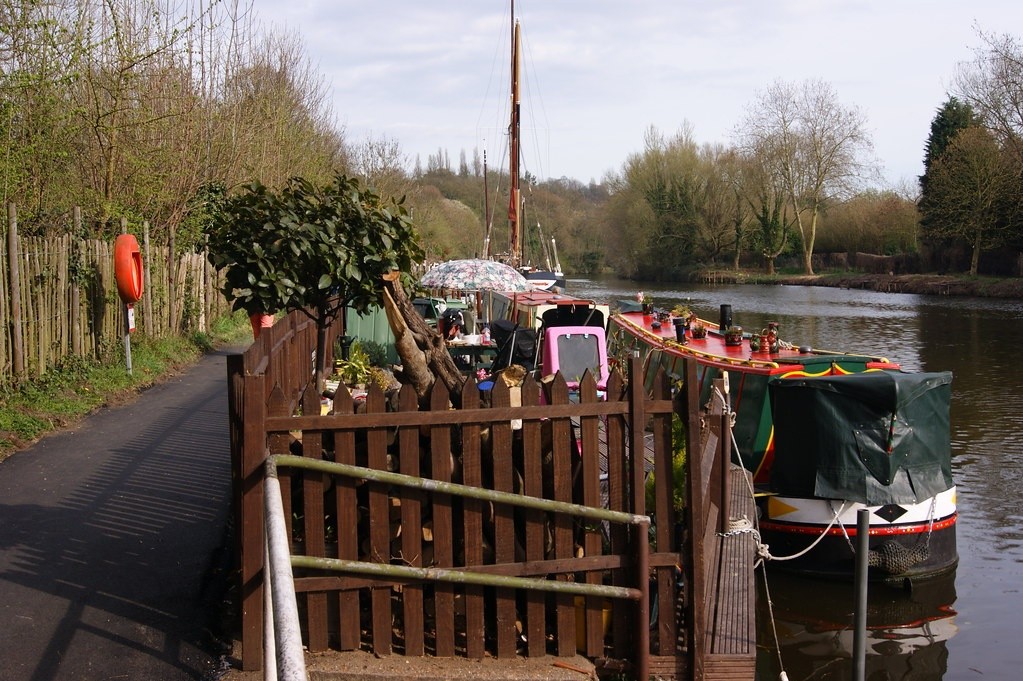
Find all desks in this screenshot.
[447,339,497,379]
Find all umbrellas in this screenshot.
[413,258,536,335]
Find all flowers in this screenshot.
[636,291,653,304]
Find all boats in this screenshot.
[550,290,961,585]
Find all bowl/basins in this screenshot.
[463,335,480,344]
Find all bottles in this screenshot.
[481,326,490,345]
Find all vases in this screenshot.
[642,304,654,315]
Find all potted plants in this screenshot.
[671,304,698,330]
[332,340,372,389]
[691,325,707,339]
[723,325,743,346]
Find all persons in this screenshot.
[444,313,473,367]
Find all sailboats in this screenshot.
[428,1,605,359]
